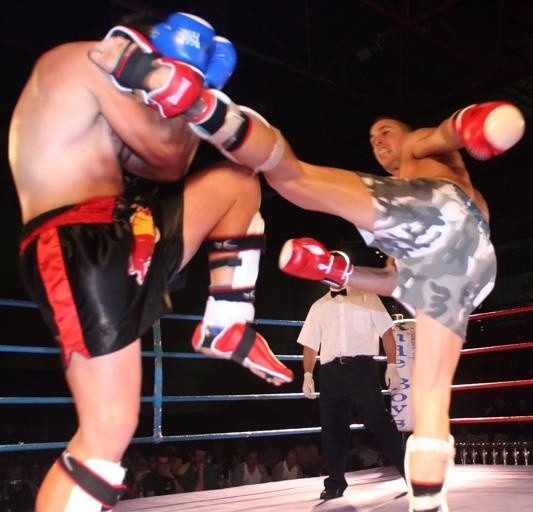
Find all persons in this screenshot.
[8,12,294,512]
[296,251,408,499]
[90,35,525,512]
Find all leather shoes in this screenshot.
[320,484,345,501]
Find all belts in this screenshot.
[327,356,375,365]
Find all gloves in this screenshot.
[149,13,214,70]
[277,236,351,291]
[205,35,237,89]
[383,363,402,390]
[451,102,526,161]
[301,371,319,399]
